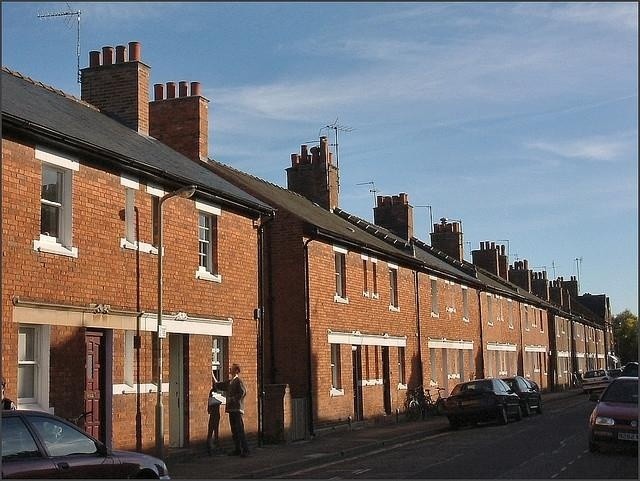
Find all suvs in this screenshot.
[499,376,543,418]
[582,361,639,392]
[443,378,523,427]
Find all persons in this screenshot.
[2,377,17,411]
[213,364,251,459]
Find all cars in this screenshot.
[0,410,169,480]
[587,377,639,454]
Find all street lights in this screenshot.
[566,316,581,389]
[155,184,199,457]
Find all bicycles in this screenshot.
[407,384,447,423]
